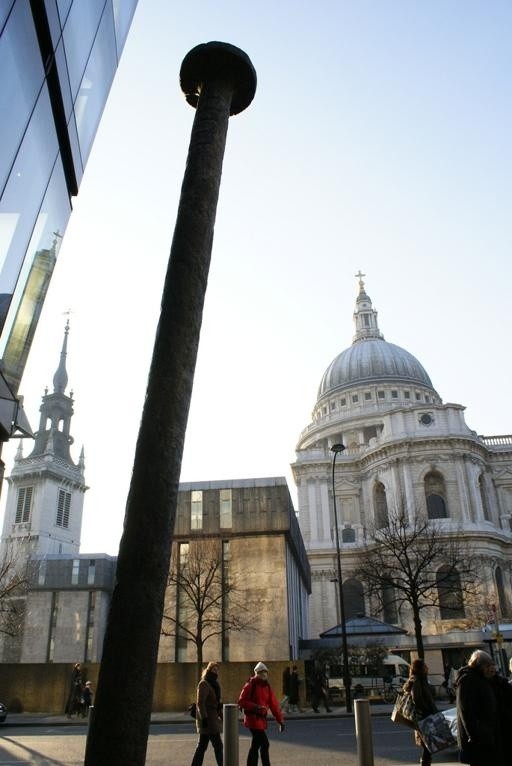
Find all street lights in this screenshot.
[330,443,354,713]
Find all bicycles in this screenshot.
[382,676,403,705]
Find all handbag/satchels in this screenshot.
[187,703,196,718]
[417,706,456,754]
[390,693,416,724]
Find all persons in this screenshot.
[391,649,512,766]
[279,665,334,714]
[189,661,286,766]
[66,663,93,721]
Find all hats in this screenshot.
[254,662,268,674]
[86,680,92,685]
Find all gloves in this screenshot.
[256,706,267,715]
[279,724,286,733]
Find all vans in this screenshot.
[325,654,436,695]
